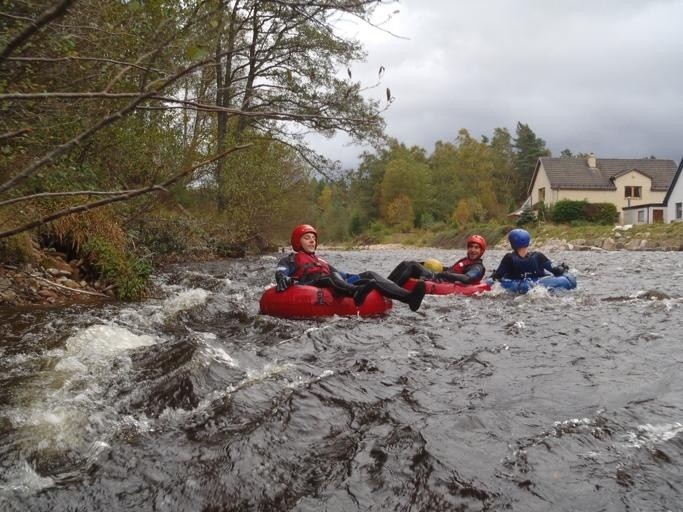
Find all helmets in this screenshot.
[466,235,486,252]
[508,229,530,249]
[424,260,443,272]
[291,225,318,251]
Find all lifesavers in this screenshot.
[401,277,490,296]
[259,282,393,318]
[500,271,577,294]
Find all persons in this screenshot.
[495,229,569,282]
[387,235,486,287]
[275,224,425,311]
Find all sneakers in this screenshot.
[353,279,376,306]
[409,282,425,311]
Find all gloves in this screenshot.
[276,273,294,292]
[435,272,450,283]
